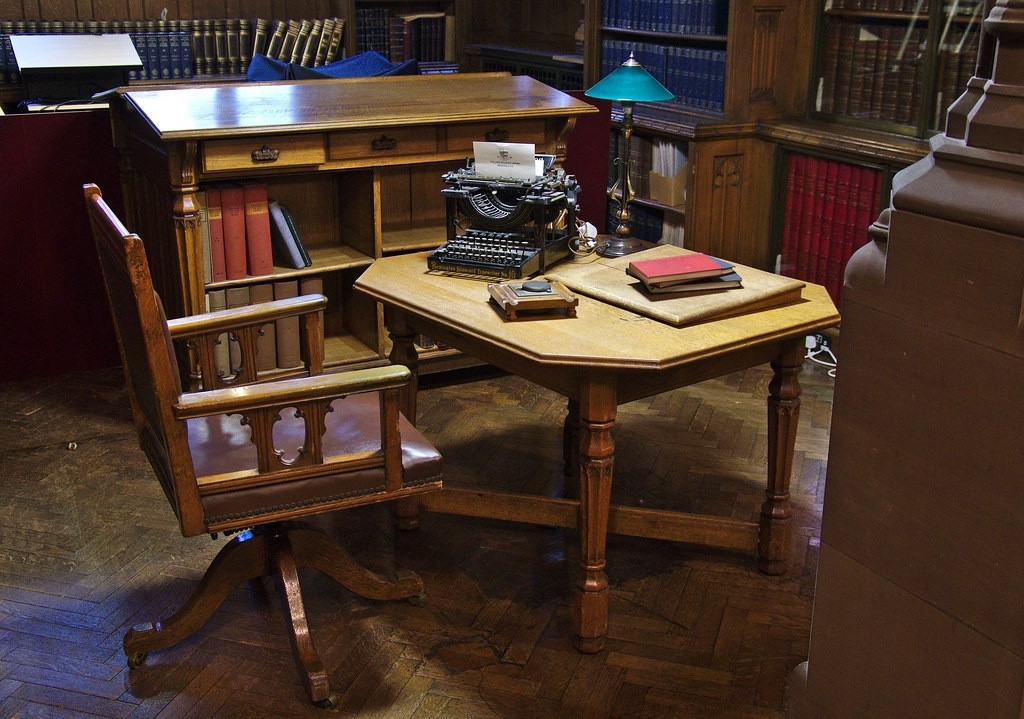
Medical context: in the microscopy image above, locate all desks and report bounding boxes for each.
[348,234,846,658]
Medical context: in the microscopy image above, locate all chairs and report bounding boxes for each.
[79,177,454,707]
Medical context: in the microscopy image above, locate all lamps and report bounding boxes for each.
[592,54,677,261]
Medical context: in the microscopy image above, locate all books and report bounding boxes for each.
[194,182,324,382]
[626,252,742,294]
[357,9,464,75]
[461,44,465,44]
[0,19,347,84]
[599,0,728,249]
[780,0,996,317]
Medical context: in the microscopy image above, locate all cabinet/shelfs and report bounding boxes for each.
[111,68,601,396]
[756,0,997,357]
[585,0,817,258]
